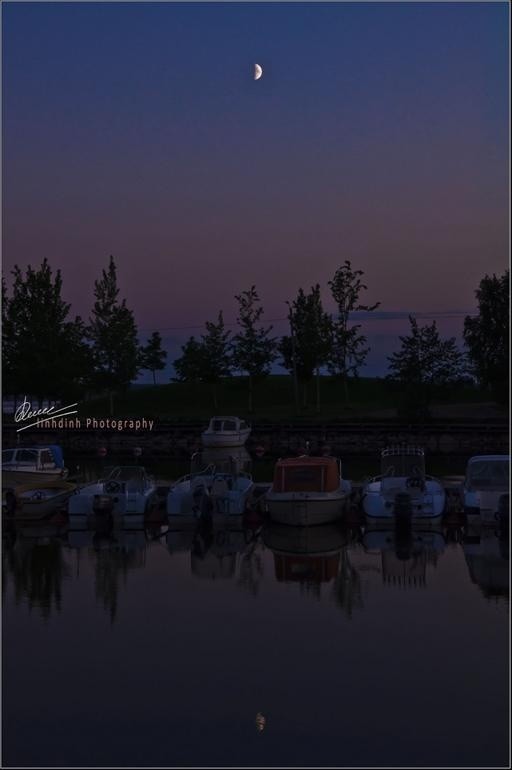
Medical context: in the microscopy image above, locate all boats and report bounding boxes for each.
[1,443,510,534]
[200,415,252,448]
[1,526,508,632]
